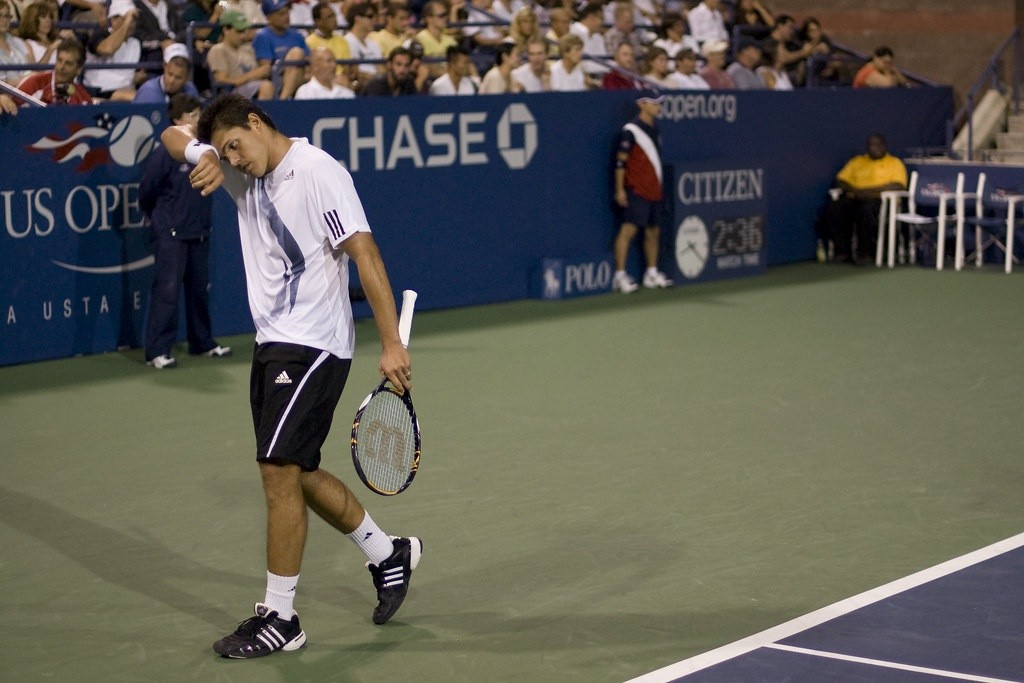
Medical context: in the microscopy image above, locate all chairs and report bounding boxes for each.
[828,169,1024,276]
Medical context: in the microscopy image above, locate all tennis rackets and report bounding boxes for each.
[351,291,420,497]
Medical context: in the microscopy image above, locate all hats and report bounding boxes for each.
[220,10,251,30]
[702,39,728,54]
[107,0,136,19]
[403,40,423,57]
[263,0,288,15]
[164,43,189,64]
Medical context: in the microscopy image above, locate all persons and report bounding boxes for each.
[834,134,908,265]
[610,87,674,293]
[0,0,909,116]
[136,94,232,371]
[161,93,422,659]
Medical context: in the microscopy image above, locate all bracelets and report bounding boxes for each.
[184,139,221,164]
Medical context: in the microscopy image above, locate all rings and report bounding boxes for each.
[405,371,410,376]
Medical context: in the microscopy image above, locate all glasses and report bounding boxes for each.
[362,13,376,20]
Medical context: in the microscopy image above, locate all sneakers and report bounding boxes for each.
[612,274,639,295]
[145,353,177,370]
[644,271,673,287]
[202,346,232,358]
[213,601,308,658]
[364,534,422,623]
